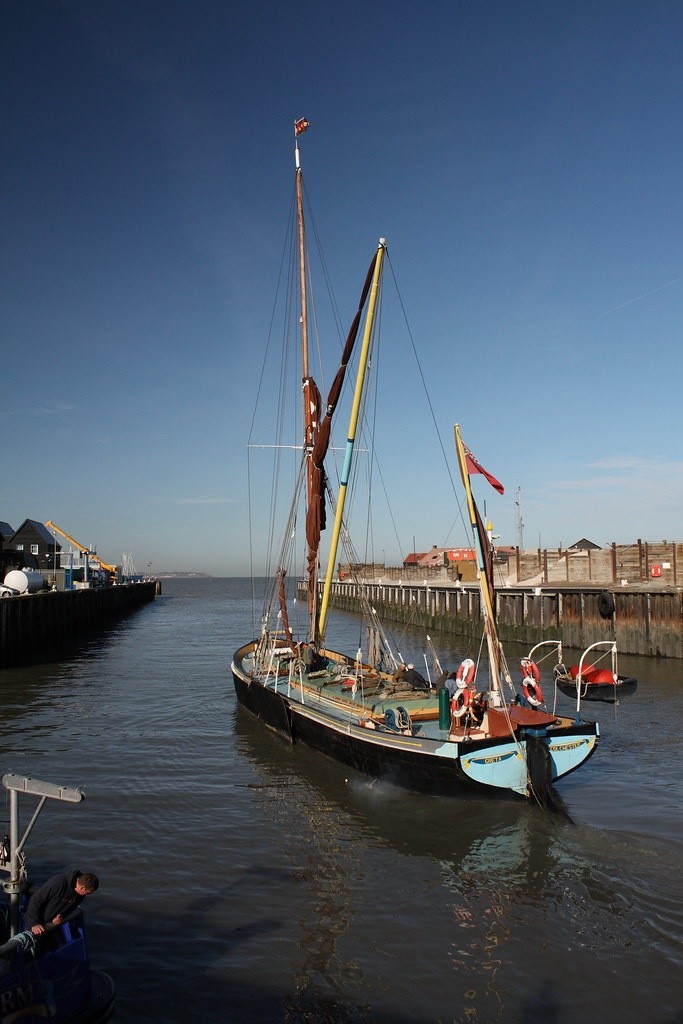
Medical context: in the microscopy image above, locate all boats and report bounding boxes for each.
[0,515,162,666]
[553,664,638,704]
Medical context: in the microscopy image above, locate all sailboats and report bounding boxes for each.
[227,115,602,805]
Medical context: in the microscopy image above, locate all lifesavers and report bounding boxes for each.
[520,656,540,685]
[522,677,543,707]
[455,659,475,687]
[450,689,470,717]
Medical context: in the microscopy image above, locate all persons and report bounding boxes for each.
[436,670,449,695]
[404,663,428,688]
[392,663,407,683]
[444,672,459,698]
[25,869,99,962]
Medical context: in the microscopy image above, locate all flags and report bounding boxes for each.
[461,440,505,495]
[294,117,307,137]
